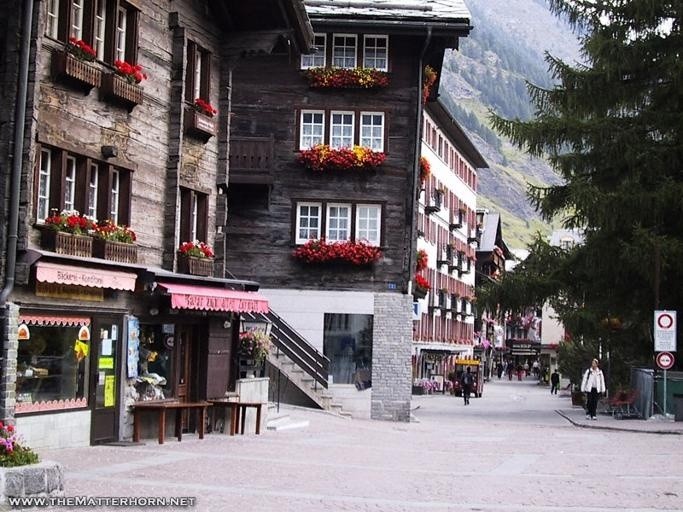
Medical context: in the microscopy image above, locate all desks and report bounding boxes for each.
[127,399,264,444]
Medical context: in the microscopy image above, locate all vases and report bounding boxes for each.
[412,284,428,298]
[185,113,215,144]
[179,255,213,277]
[40,228,137,266]
[105,74,142,106]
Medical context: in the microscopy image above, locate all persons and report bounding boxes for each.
[459,365,475,405]
[549,368,559,394]
[580,357,606,420]
[495,362,503,380]
[517,366,523,381]
[523,359,530,376]
[508,363,513,381]
[532,359,538,376]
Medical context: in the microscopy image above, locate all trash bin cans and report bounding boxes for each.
[629,367,683,422]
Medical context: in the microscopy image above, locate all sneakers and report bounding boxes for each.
[467,399,469,405]
[584,415,597,420]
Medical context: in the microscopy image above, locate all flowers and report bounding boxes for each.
[238,326,273,362]
[35,205,136,245]
[187,97,218,118]
[60,37,143,88]
[417,156,431,187]
[307,66,391,90]
[413,249,431,289]
[289,239,385,267]
[178,240,215,258]
[297,140,385,174]
[421,64,436,104]
